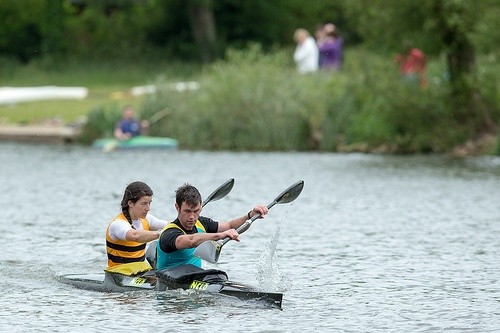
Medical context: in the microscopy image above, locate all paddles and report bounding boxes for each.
[103,106,173,152]
[194,179,304,263]
[144,179,236,258]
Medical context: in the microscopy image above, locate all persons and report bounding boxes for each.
[394,48,428,88]
[105,181,173,280]
[292,23,343,76]
[113,105,149,141]
[155,183,270,277]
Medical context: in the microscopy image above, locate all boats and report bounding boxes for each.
[54,268,284,310]
[92,136,179,155]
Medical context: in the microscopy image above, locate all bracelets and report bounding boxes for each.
[248,210,252,219]
